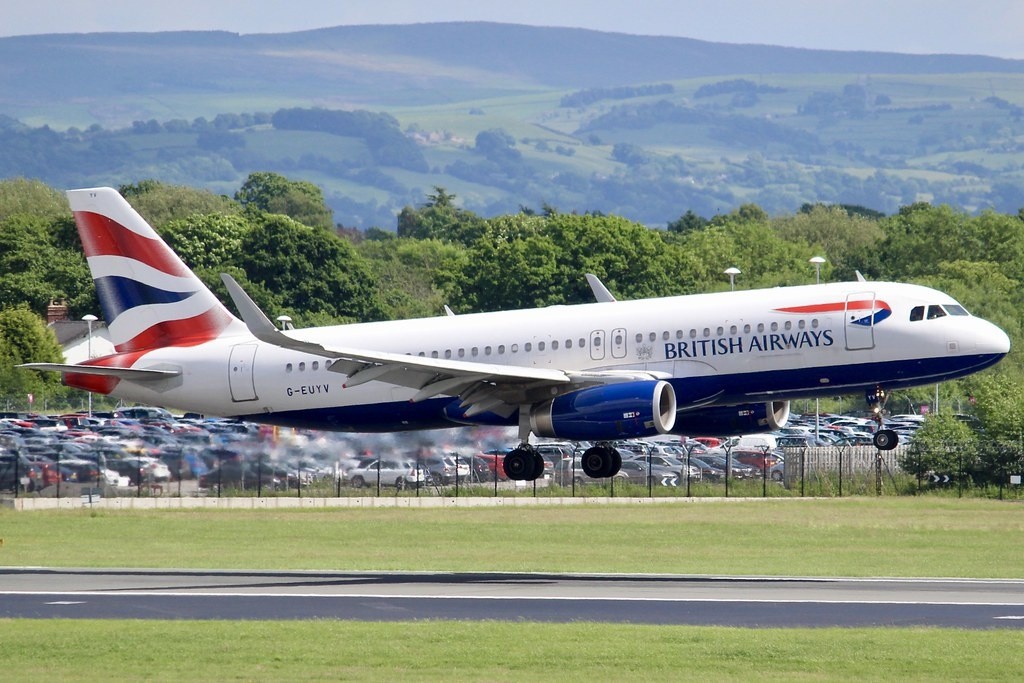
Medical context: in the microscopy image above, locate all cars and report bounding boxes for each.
[0,406,989,490]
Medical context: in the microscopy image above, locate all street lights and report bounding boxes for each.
[809,257,827,284]
[724,268,741,292]
[81,314,98,417]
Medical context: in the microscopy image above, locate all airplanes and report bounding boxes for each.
[15,186,1010,481]
